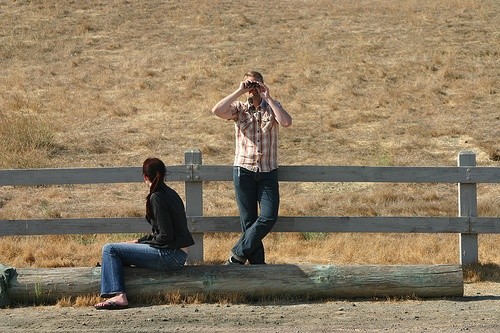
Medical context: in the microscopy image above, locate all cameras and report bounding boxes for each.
[248,82,260,88]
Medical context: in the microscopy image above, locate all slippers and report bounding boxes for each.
[94,300,129,310]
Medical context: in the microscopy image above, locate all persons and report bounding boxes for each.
[93,157,196,309]
[212,70,293,266]
